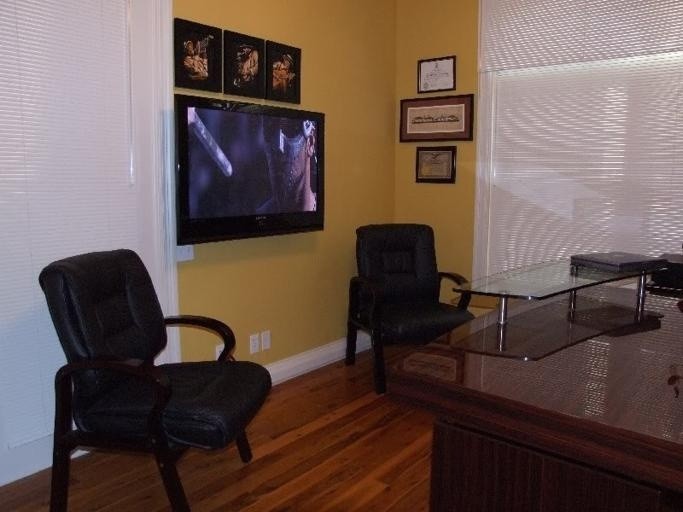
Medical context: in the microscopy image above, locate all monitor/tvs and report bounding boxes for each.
[174,94,324,247]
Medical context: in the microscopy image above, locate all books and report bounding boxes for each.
[570,248,667,272]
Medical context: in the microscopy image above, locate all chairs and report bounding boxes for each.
[345,223,472,394]
[38,246,274,512]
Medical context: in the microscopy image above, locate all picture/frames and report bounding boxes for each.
[174,18,223,92]
[397,94,474,142]
[263,38,301,104]
[417,54,457,93]
[222,28,264,100]
[415,145,456,183]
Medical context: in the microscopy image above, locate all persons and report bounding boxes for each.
[232,42,259,88]
[182,40,209,81]
[256,116,317,214]
[271,53,296,96]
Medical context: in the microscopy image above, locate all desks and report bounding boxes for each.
[398,271,683,510]
